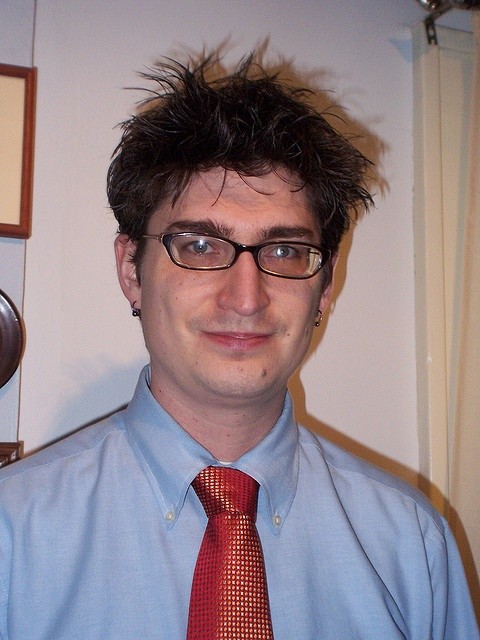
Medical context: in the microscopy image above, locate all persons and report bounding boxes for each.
[0,55,477,639]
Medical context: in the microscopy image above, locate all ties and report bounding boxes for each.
[186,465,274,640]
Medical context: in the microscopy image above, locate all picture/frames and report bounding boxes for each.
[0,61,38,239]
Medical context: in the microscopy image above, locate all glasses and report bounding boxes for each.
[139,232,331,279]
